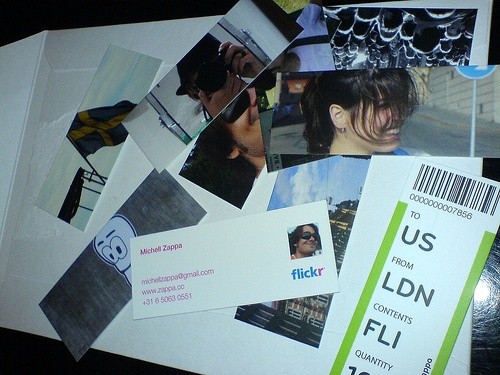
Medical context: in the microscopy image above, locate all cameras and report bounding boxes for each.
[187,46,244,98]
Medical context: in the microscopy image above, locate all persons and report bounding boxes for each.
[287,222,322,260]
[300,67,413,155]
[176,33,266,137]
[198,81,267,176]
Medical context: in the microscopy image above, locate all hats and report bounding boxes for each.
[176,33,223,96]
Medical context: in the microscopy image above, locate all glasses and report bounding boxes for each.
[301,232,319,241]
[220,69,268,122]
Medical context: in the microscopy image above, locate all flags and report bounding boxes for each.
[66,100,137,160]
